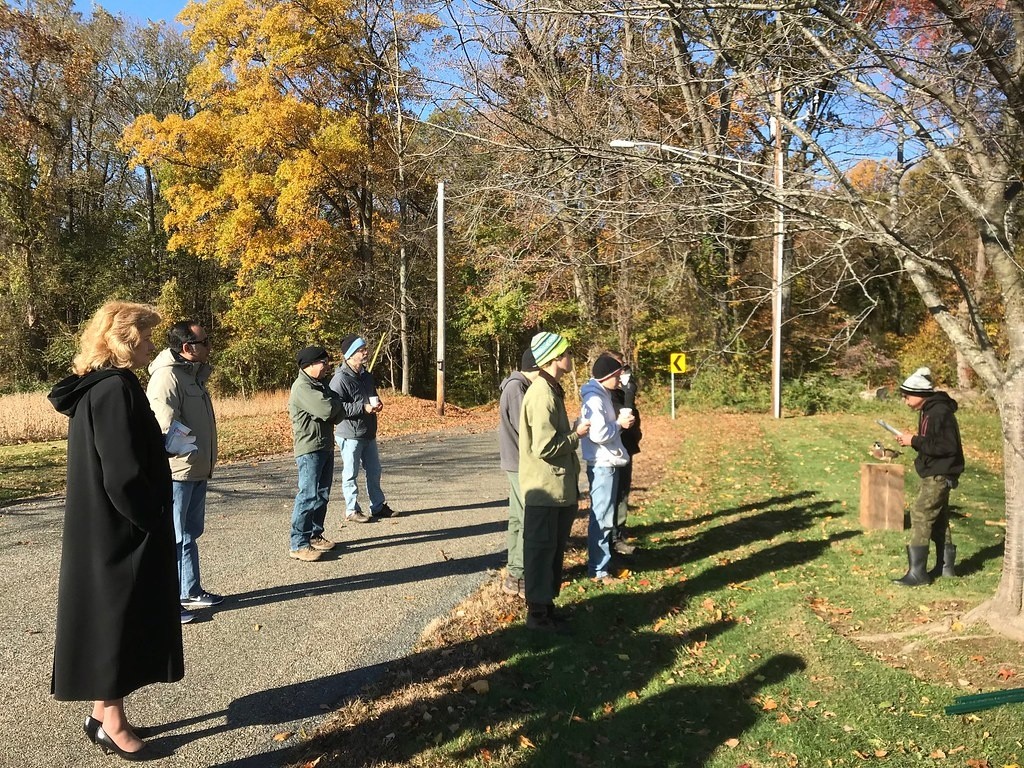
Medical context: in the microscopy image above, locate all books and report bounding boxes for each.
[875,419,903,437]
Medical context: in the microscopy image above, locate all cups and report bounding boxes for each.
[165,419,192,450]
[619,407,632,416]
[369,396,378,406]
[619,374,631,386]
[577,417,590,428]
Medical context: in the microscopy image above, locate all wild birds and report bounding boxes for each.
[873,441,904,464]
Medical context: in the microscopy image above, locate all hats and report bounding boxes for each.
[899,366,935,397]
[341,333,366,360]
[521,347,540,371]
[531,332,569,368]
[592,356,622,381]
[297,347,328,369]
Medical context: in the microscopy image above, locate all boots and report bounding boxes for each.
[891,544,933,587]
[927,544,960,580]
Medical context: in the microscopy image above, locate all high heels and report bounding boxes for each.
[83,715,103,745]
[95,724,162,762]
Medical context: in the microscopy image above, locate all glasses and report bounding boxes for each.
[186,337,210,347]
[316,360,328,365]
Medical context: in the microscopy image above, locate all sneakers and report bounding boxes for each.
[180,589,224,606]
[180,603,197,624]
[347,510,371,523]
[309,534,336,550]
[289,545,323,562]
[372,502,399,517]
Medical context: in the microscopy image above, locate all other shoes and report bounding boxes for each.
[611,538,638,556]
[590,574,624,587]
[518,578,525,599]
[524,611,566,633]
[550,603,570,621]
[501,570,519,595]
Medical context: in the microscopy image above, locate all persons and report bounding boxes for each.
[612,359,642,544]
[581,355,637,578]
[146,321,225,623]
[289,348,346,562]
[48,301,185,760]
[520,332,592,628]
[329,334,400,523]
[498,348,540,600]
[893,367,965,586]
